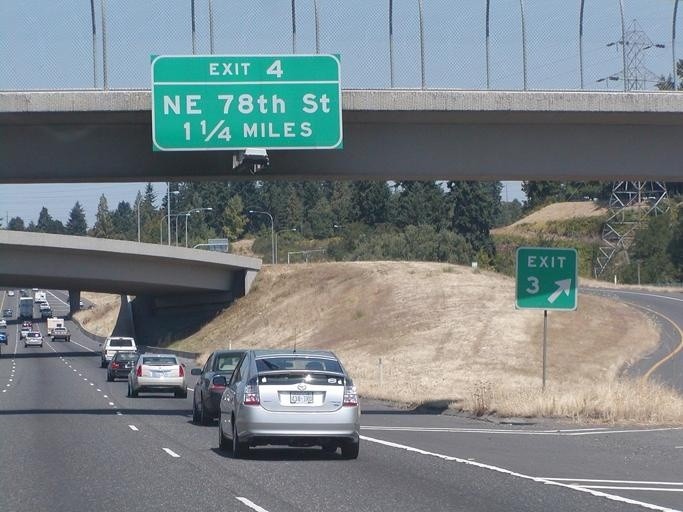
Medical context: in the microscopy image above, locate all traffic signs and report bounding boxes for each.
[151,56,342,153]
[515,246,576,310]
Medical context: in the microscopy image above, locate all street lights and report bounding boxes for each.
[334,224,345,229]
[135,189,309,266]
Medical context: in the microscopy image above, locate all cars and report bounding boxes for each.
[217,348,360,461]
[191,347,243,424]
[0,286,187,398]
